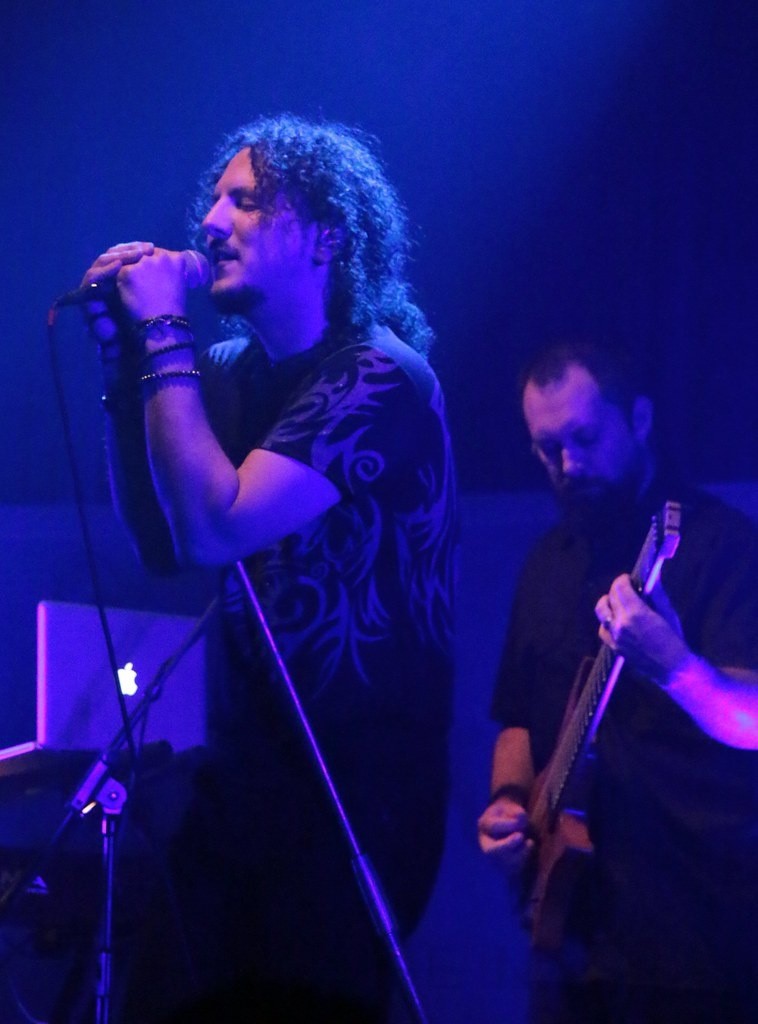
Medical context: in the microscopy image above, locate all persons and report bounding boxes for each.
[477,327,758,1023]
[76,113,458,1024]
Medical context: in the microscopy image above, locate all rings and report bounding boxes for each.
[603,617,612,630]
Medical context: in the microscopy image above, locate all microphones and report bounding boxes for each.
[56,250,210,306]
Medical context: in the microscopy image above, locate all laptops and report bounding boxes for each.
[0,601,204,776]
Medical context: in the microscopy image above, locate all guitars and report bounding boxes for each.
[512,496,683,955]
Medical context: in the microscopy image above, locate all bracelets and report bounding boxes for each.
[138,340,192,362]
[140,367,202,380]
[135,313,192,331]
[489,783,530,809]
[96,337,139,413]
